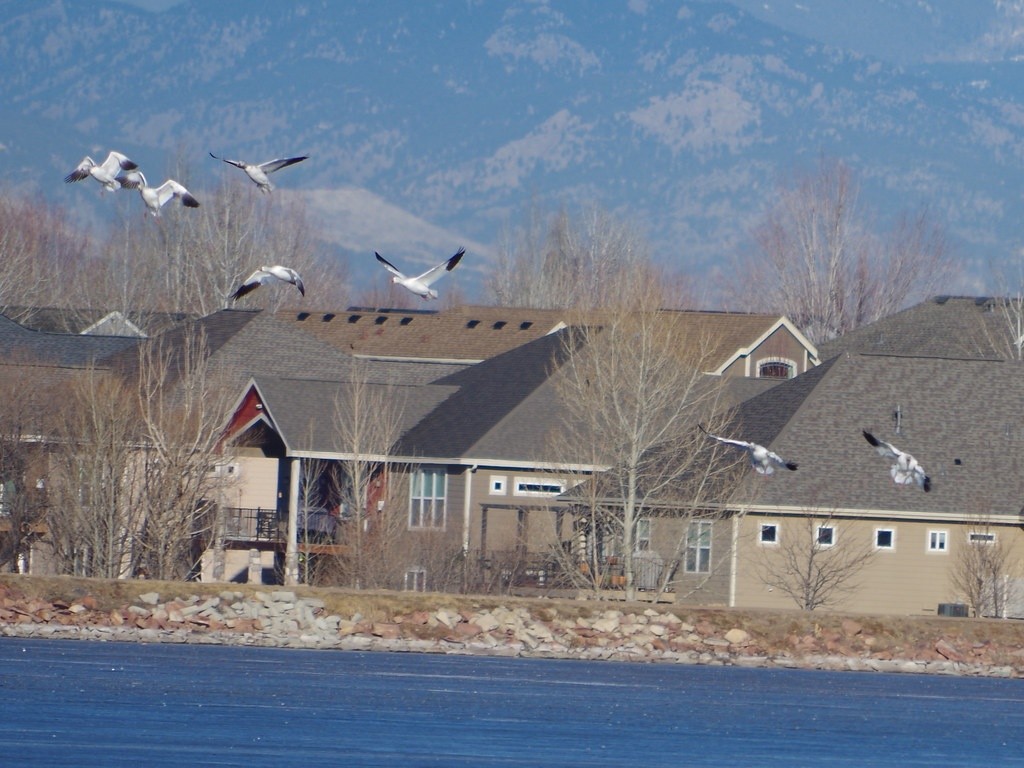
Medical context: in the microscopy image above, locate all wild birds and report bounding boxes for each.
[230,265,304,301]
[207,150,310,195]
[63,151,138,191]
[115,172,200,218]
[695,422,800,476]
[861,430,931,494]
[373,246,467,301]
